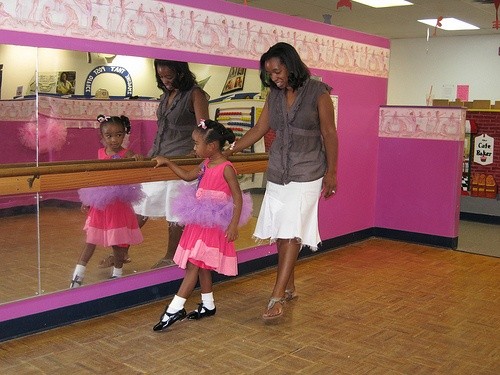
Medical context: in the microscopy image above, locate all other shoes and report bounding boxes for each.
[153,306,187,332]
[187,303,216,320]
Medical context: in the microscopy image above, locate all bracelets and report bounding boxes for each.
[228,140,237,157]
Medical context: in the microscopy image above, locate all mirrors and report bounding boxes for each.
[0,44,322,306]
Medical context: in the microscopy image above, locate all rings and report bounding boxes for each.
[331,189,336,194]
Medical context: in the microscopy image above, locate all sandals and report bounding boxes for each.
[98,254,131,268]
[285,286,296,300]
[149,259,174,269]
[263,297,285,321]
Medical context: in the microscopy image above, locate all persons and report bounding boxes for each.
[97,59,210,282]
[224,68,244,90]
[151,118,255,332]
[57,72,72,95]
[70,113,145,291]
[219,42,339,321]
